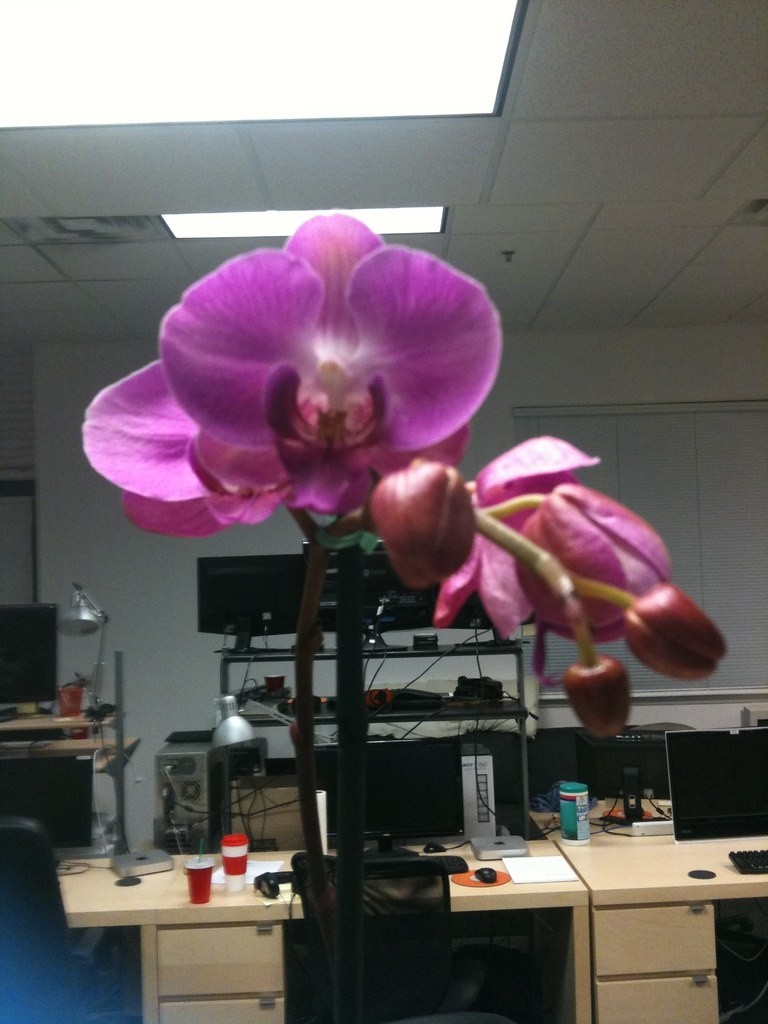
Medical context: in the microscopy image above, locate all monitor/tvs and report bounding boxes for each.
[1,756,93,846]
[196,541,536,652]
[0,603,59,704]
[312,737,465,860]
[664,726,768,844]
[576,729,671,826]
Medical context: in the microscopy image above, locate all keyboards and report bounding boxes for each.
[729,849,768,875]
[364,856,468,880]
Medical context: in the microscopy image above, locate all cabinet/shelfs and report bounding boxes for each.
[212,640,533,840]
[1,710,141,857]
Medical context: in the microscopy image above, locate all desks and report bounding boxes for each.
[529,795,768,1024]
[52,839,594,1024]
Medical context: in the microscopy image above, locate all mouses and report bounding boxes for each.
[475,867,497,883]
[423,841,446,853]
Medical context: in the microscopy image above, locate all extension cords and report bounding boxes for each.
[632,821,674,835]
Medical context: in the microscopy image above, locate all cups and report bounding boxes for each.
[264,676,285,690]
[221,834,249,891]
[185,857,215,904]
[60,689,82,716]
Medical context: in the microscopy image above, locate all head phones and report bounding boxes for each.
[255,871,301,898]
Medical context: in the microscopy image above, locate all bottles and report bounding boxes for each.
[560,783,591,846]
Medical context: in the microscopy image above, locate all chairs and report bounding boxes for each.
[0,820,107,1024]
[522,727,625,814]
[288,850,549,1024]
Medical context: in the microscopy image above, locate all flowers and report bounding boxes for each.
[85,208,732,740]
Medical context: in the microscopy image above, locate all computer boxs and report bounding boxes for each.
[154,740,223,855]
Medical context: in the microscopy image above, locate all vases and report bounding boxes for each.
[56,687,83,718]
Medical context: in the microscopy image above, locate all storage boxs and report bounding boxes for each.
[228,773,309,852]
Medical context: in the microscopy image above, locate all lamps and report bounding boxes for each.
[57,580,109,703]
